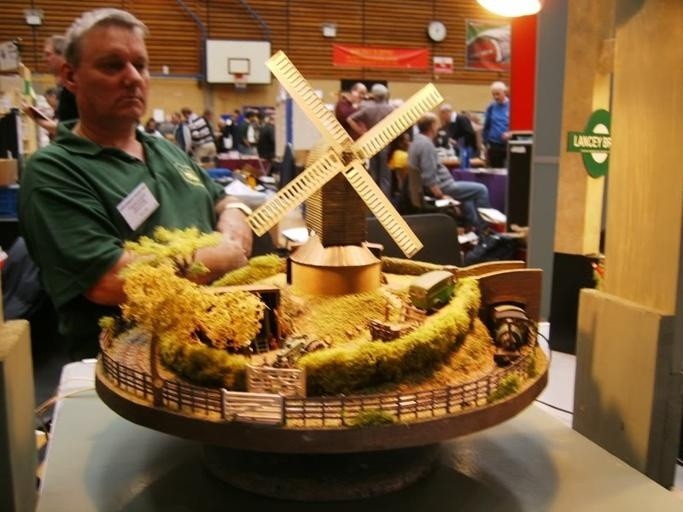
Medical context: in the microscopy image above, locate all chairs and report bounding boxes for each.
[403,165,462,222]
[353,214,461,265]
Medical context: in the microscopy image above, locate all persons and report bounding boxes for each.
[21,34,78,146]
[18,8,253,362]
[336,78,512,239]
[143,101,276,178]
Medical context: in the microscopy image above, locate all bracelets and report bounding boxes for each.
[224,202,253,217]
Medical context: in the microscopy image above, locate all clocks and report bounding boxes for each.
[427,21,447,44]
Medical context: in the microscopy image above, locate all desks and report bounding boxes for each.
[452,167,507,208]
[36,357,681,510]
[212,152,257,170]
